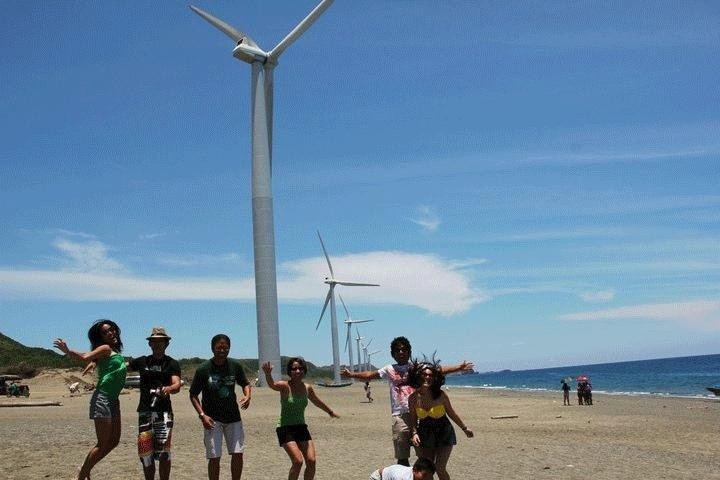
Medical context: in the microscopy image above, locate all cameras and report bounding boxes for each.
[149,386,164,408]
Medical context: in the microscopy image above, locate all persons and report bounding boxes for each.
[123,327,182,480]
[369,457,436,480]
[407,349,474,480]
[363,382,373,403]
[561,379,592,406]
[339,336,476,468]
[189,334,252,480]
[51,318,127,480]
[261,357,340,480]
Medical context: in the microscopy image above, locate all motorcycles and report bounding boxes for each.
[6,388,20,398]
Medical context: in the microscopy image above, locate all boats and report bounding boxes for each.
[315,382,354,388]
[707,385,720,397]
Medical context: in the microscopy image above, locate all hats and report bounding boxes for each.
[145,327,172,341]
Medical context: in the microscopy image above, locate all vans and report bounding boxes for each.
[125,375,141,389]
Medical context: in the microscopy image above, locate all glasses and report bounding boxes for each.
[290,366,304,371]
[394,346,409,353]
[100,325,116,336]
[419,371,434,378]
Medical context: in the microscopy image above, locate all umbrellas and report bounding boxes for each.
[576,375,591,382]
[562,376,573,382]
